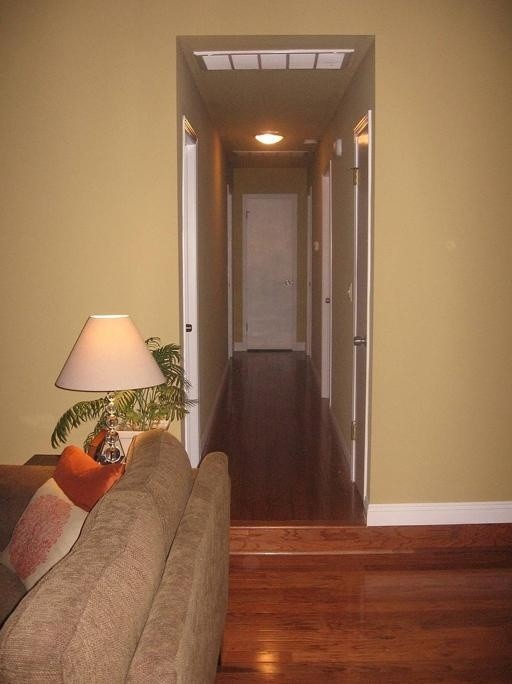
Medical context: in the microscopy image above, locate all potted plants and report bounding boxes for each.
[51,336,200,458]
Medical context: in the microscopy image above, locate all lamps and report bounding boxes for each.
[55,314,167,462]
[255,133,284,144]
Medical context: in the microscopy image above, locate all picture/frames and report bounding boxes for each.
[88,430,107,460]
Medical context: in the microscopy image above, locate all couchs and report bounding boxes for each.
[0,428,231,683]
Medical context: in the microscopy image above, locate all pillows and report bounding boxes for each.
[0,444,125,593]
